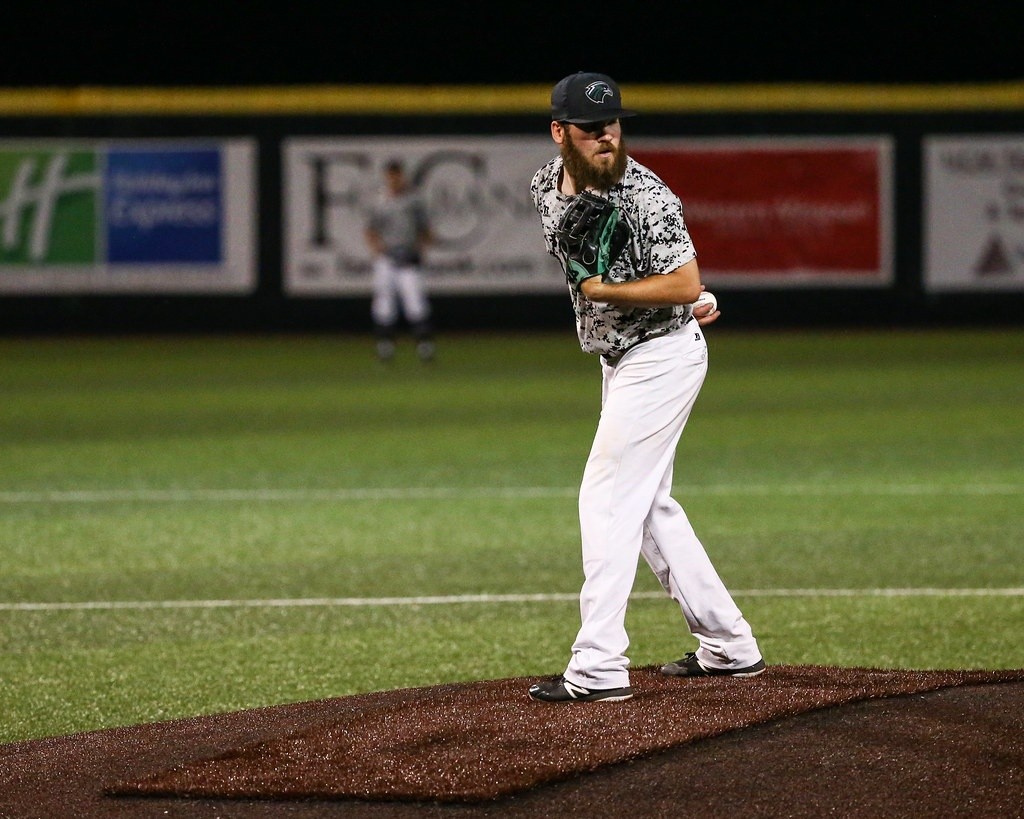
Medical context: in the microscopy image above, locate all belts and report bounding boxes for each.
[601,332,663,359]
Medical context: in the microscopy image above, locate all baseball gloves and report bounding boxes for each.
[556,191,631,290]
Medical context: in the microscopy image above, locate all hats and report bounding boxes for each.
[551,71,637,124]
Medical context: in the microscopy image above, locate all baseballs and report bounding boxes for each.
[691,291,718,317]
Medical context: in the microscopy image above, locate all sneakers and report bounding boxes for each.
[528,673,634,702]
[660,651,766,678]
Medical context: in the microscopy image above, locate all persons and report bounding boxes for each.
[361,160,444,356]
[528,73,764,704]
